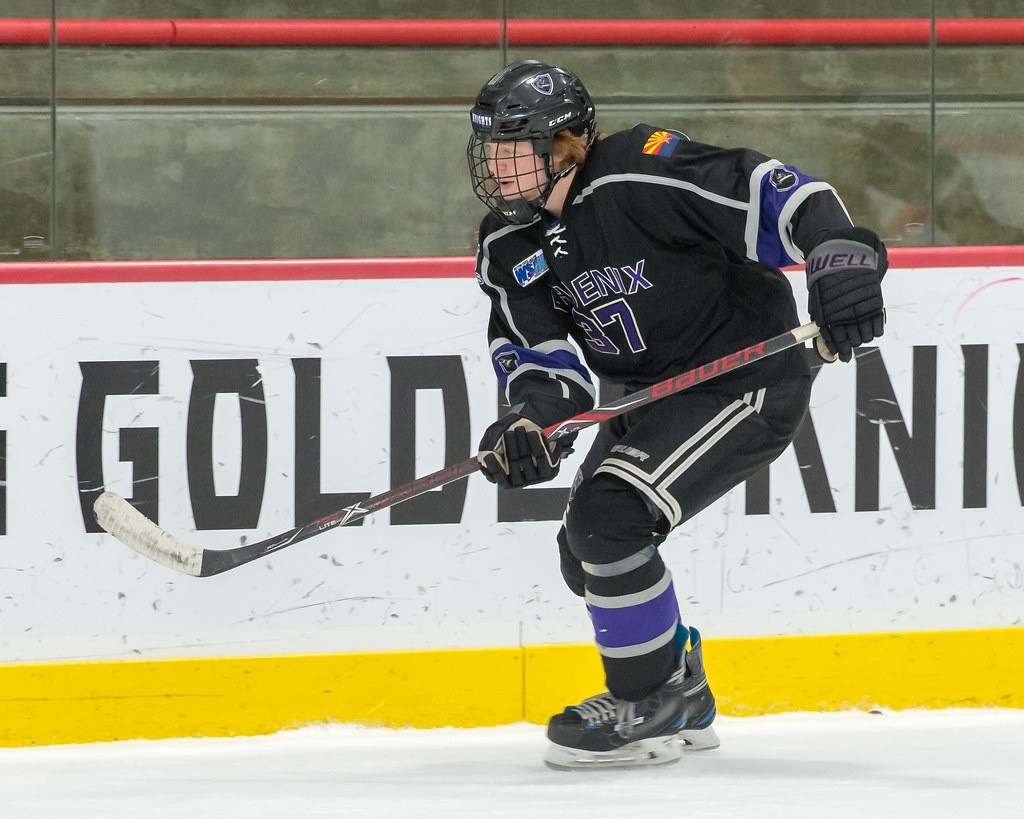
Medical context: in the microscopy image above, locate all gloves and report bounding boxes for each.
[477,390,580,491]
[804,226,890,364]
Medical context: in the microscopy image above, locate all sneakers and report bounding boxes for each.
[542,625,720,770]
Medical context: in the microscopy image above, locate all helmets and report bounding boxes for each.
[466,59,597,226]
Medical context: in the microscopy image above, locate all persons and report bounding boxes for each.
[465,59,889,771]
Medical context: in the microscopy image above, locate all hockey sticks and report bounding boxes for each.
[94,320,821,579]
